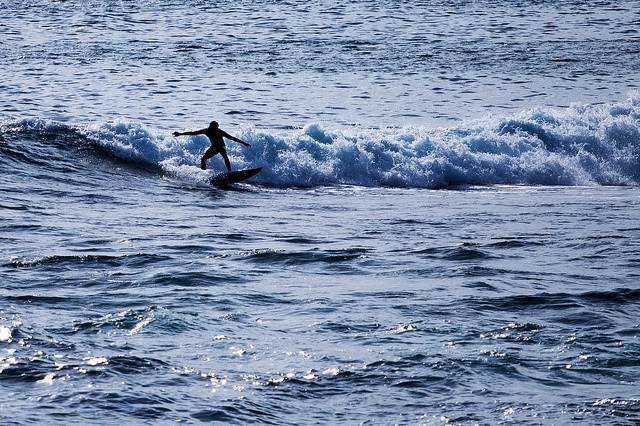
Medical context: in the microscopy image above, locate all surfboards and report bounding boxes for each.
[210,167,263,186]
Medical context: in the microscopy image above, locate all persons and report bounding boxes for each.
[172,121,251,172]
